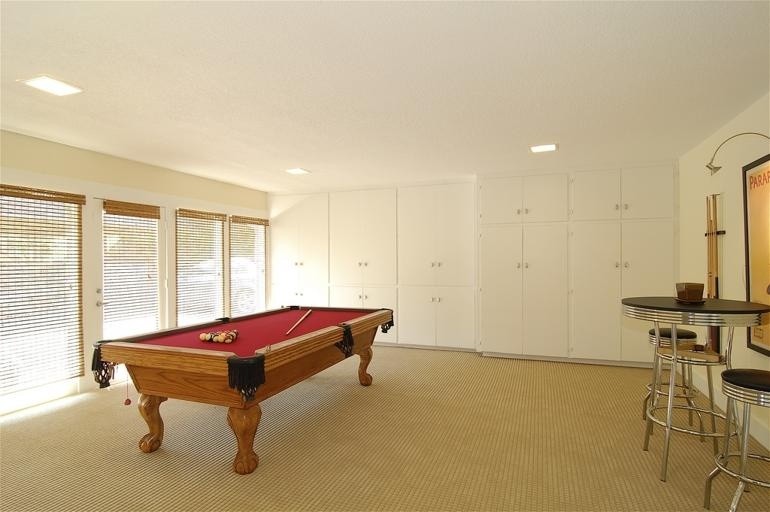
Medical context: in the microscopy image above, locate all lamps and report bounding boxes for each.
[704,132,770,176]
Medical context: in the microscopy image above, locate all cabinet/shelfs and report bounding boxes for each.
[396,179,479,287]
[567,217,682,368]
[264,191,328,284]
[328,283,396,349]
[477,169,570,226]
[566,159,681,220]
[475,220,569,365]
[327,187,398,287]
[267,283,331,311]
[396,283,480,354]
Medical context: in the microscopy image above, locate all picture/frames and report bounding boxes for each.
[739,153,769,358]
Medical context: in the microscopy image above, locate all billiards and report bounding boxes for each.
[198,330,239,344]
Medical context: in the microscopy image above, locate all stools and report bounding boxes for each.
[639,326,708,447]
[700,362,769,512]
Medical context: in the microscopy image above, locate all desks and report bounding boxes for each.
[619,292,769,495]
[87,304,399,477]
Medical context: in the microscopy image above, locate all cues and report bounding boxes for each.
[285,309,312,333]
[702,193,717,357]
